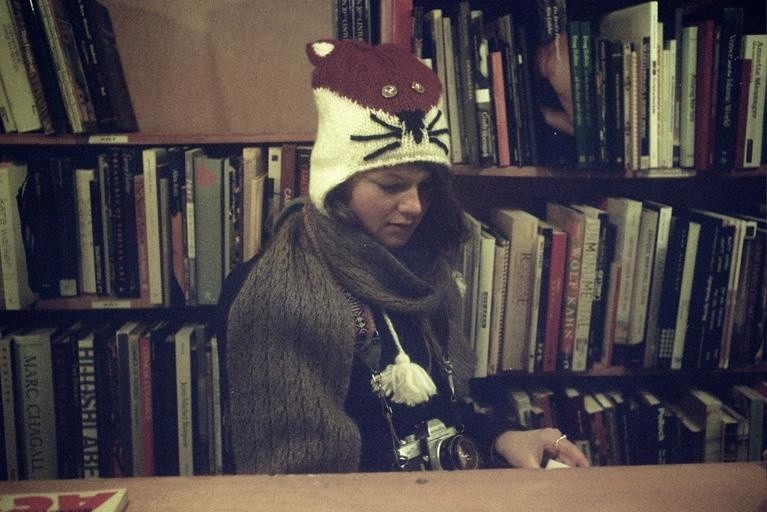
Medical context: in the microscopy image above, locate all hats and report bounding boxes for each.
[306,39,467,407]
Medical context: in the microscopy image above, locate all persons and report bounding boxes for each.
[216,39,595,475]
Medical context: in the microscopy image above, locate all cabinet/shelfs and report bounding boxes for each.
[3,4,765,382]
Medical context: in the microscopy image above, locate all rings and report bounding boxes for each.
[556,434,567,448]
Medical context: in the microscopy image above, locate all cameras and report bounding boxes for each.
[393,417,480,470]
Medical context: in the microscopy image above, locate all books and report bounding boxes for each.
[332,2,765,170]
[506,385,766,467]
[2,1,140,135]
[1,314,237,482]
[457,197,766,379]
[0,489,129,512]
[0,146,309,310]
